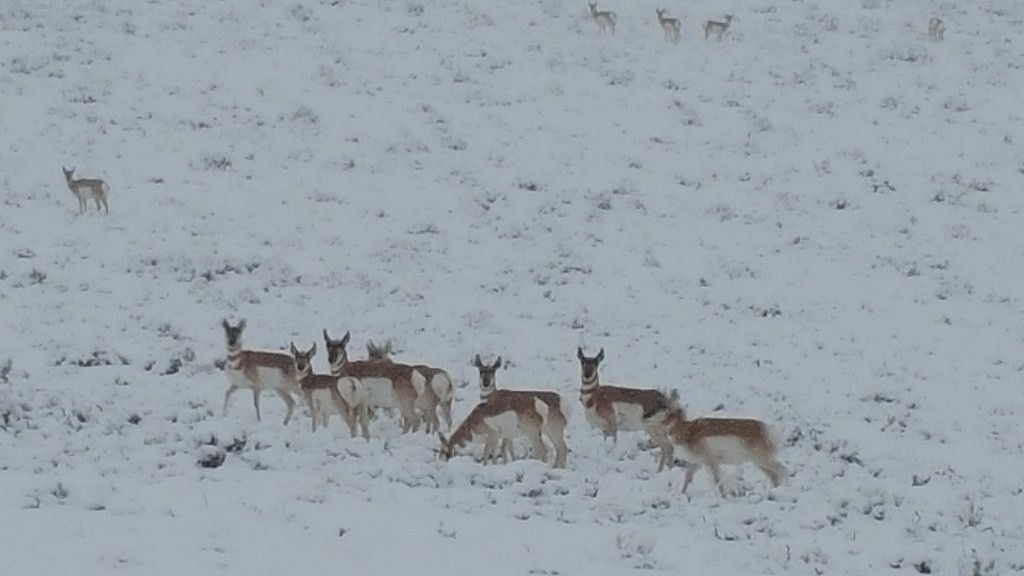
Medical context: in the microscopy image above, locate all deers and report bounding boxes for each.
[62,166,110,214]
[588,1,617,34]
[704,14,736,41]
[437,354,569,469]
[655,8,683,46]
[641,388,783,500]
[577,347,683,476]
[221,320,452,443]
